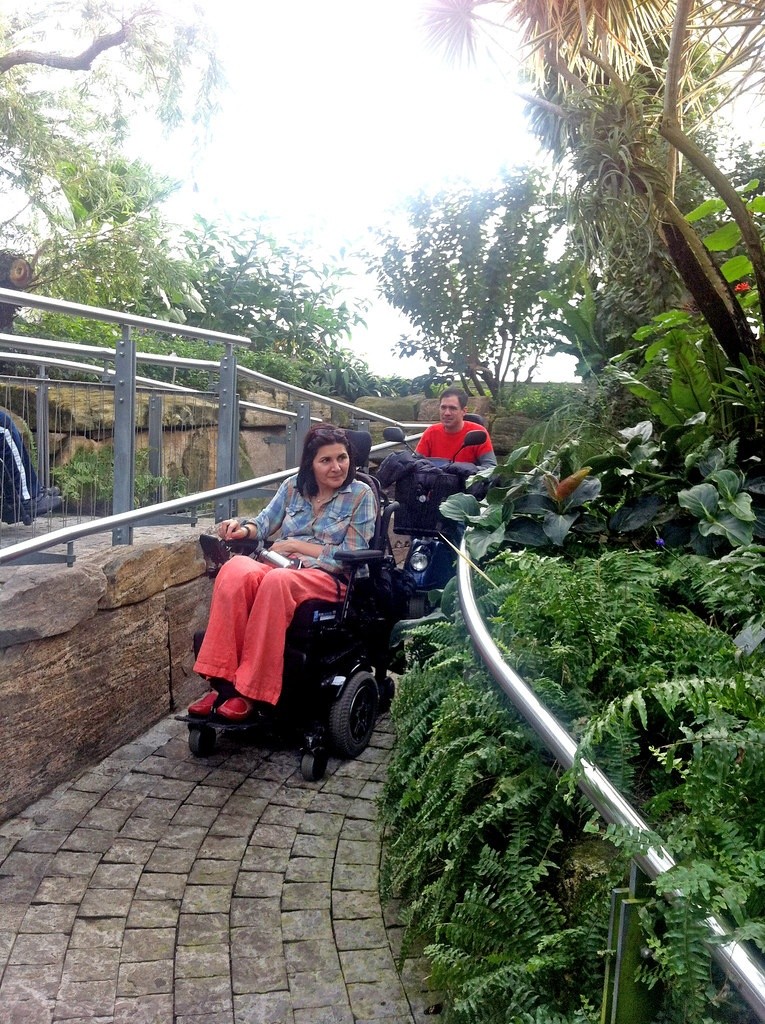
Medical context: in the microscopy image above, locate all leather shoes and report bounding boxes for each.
[216,697,252,719]
[187,692,217,714]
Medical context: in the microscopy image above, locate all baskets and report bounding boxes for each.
[392,473,460,540]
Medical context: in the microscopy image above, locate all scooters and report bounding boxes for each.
[382,414,487,618]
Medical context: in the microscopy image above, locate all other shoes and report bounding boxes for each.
[22,494,63,516]
[40,486,59,496]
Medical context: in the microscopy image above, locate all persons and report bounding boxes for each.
[411,387,497,592]
[187,422,379,719]
[0,411,64,523]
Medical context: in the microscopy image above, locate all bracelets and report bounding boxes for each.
[235,524,252,540]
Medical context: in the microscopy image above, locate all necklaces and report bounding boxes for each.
[314,493,331,504]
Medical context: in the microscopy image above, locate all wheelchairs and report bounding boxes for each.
[174,431,430,783]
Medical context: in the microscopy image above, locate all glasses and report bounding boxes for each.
[306,428,346,446]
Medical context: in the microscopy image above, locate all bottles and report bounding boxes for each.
[255,547,294,568]
